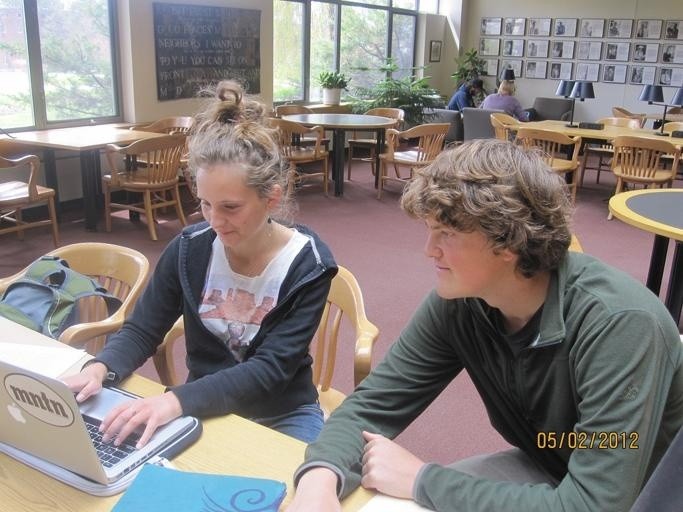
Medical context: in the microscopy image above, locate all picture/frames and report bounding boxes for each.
[477,17,683,88]
[430,40,442,62]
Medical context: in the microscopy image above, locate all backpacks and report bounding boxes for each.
[0,255,123,341]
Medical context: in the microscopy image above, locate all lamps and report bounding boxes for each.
[638,84,683,136]
[555,80,595,128]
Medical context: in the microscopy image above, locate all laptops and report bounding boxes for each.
[0,361,195,484]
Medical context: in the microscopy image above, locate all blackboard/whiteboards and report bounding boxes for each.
[153,4,261,101]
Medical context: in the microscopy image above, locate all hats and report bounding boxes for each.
[470,79,487,91]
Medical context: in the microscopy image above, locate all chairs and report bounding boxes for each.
[277,106,330,180]
[376,122,451,200]
[422,97,683,221]
[0,155,61,249]
[347,108,405,180]
[130,117,198,214]
[153,264,380,423]
[102,134,188,241]
[263,118,329,204]
[0,242,151,357]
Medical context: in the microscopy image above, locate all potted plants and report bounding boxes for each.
[320,72,352,105]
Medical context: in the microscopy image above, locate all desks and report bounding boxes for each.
[609,188,683,334]
[0,373,379,512]
[280,114,398,197]
[0,123,170,232]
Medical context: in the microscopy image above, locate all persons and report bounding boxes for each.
[447,78,483,116]
[283,136,682,511]
[478,78,529,122]
[64,78,339,450]
